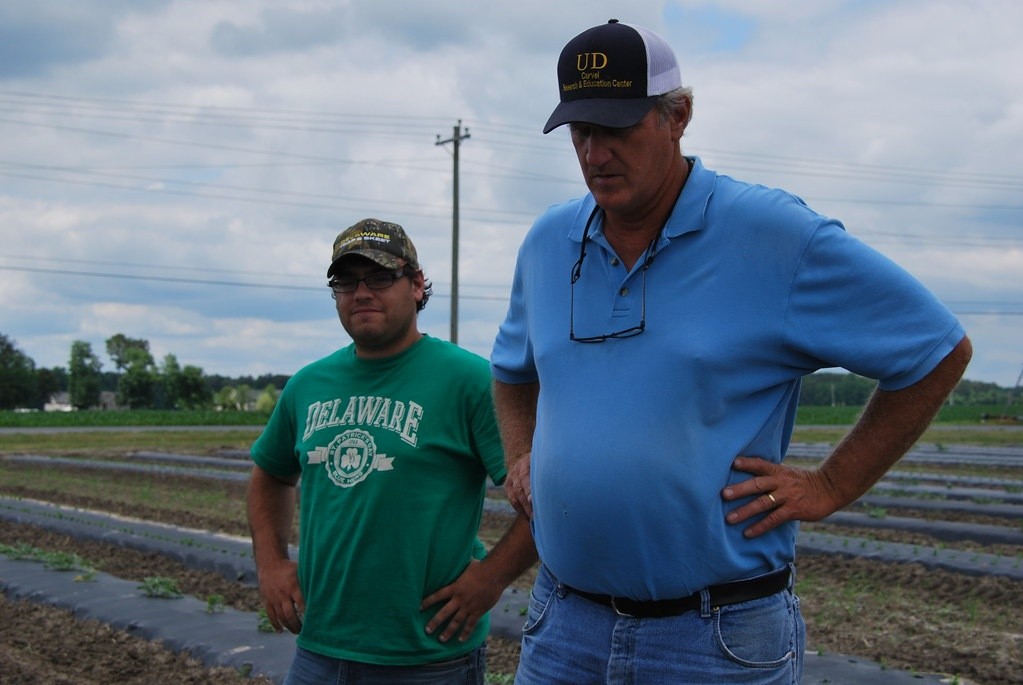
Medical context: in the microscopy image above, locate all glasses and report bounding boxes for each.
[329,270,406,294]
[569,237,657,344]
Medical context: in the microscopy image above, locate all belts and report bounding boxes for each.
[563,563,792,620]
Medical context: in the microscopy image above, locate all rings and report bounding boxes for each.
[768,493,776,508]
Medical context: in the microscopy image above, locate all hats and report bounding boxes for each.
[326,218,420,279]
[542,18,682,135]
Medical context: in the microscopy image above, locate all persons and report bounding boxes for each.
[490,19,972,685]
[247,219,539,685]
